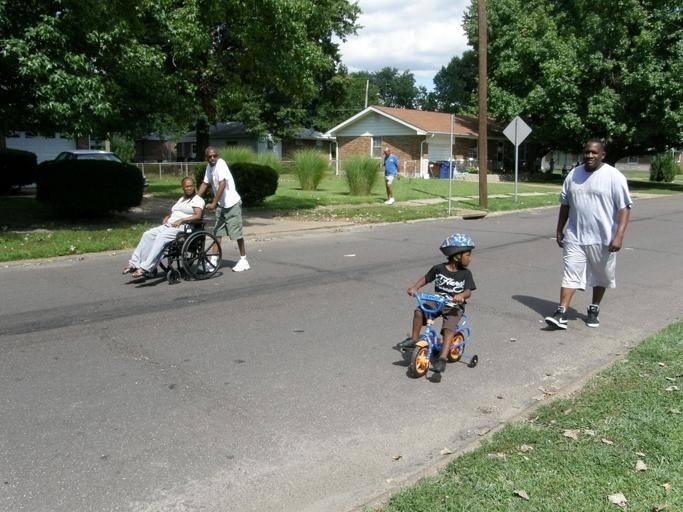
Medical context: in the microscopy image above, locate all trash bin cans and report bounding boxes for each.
[428,160,455,179]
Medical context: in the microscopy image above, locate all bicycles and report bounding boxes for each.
[400,290,478,378]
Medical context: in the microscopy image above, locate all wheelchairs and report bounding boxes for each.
[130,205,223,284]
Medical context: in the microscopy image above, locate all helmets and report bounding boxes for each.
[439,232,475,257]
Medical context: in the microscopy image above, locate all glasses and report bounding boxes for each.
[208,153,218,158]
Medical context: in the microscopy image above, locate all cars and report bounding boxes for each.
[55,150,150,191]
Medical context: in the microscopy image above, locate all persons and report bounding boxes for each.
[120,177,206,280]
[401,233,475,374]
[196,146,252,273]
[380,148,401,205]
[544,140,632,331]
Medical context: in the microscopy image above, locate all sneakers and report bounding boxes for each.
[198,262,218,272]
[123,265,134,274]
[397,337,417,348]
[385,197,394,205]
[545,306,568,329]
[232,260,251,272]
[429,358,447,372]
[132,267,148,278]
[586,304,600,327]
